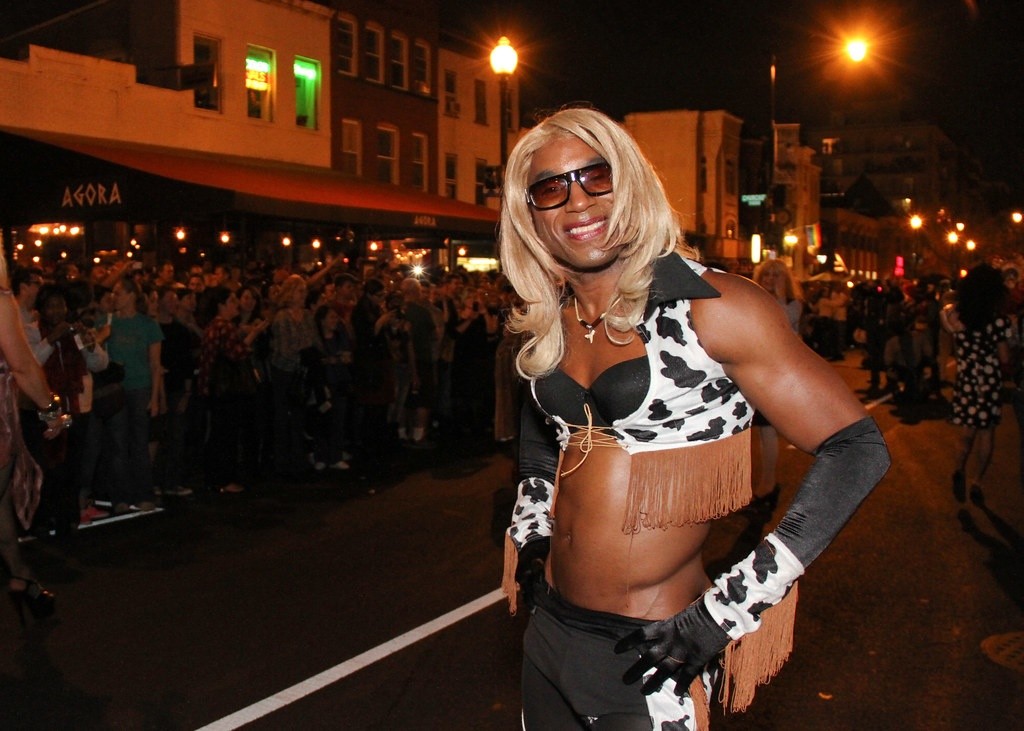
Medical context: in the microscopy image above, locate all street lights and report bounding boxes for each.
[486,34,521,177]
[767,31,867,265]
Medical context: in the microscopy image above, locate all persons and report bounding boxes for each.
[43,233,1024,635]
[0,286,56,629]
[499,107,892,731]
[11,268,43,306]
[24,282,110,526]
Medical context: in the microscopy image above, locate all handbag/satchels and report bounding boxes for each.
[77,374,93,413]
[81,332,109,372]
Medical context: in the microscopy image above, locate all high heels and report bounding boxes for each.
[8,576,57,628]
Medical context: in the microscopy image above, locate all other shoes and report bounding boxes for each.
[315,463,325,470]
[166,488,192,496]
[329,460,349,469]
[140,502,154,511]
[412,428,425,441]
[79,507,109,525]
[969,485,983,504]
[227,484,243,492]
[397,428,406,439]
[115,503,129,513]
[953,470,966,502]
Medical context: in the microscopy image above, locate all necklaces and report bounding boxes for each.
[574,296,621,344]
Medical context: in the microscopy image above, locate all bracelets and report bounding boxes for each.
[37,392,62,422]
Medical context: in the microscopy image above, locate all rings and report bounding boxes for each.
[47,427,56,432]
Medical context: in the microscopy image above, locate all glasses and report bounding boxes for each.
[375,293,385,298]
[524,162,613,211]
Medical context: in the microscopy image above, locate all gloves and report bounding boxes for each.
[515,536,550,610]
[613,587,732,696]
